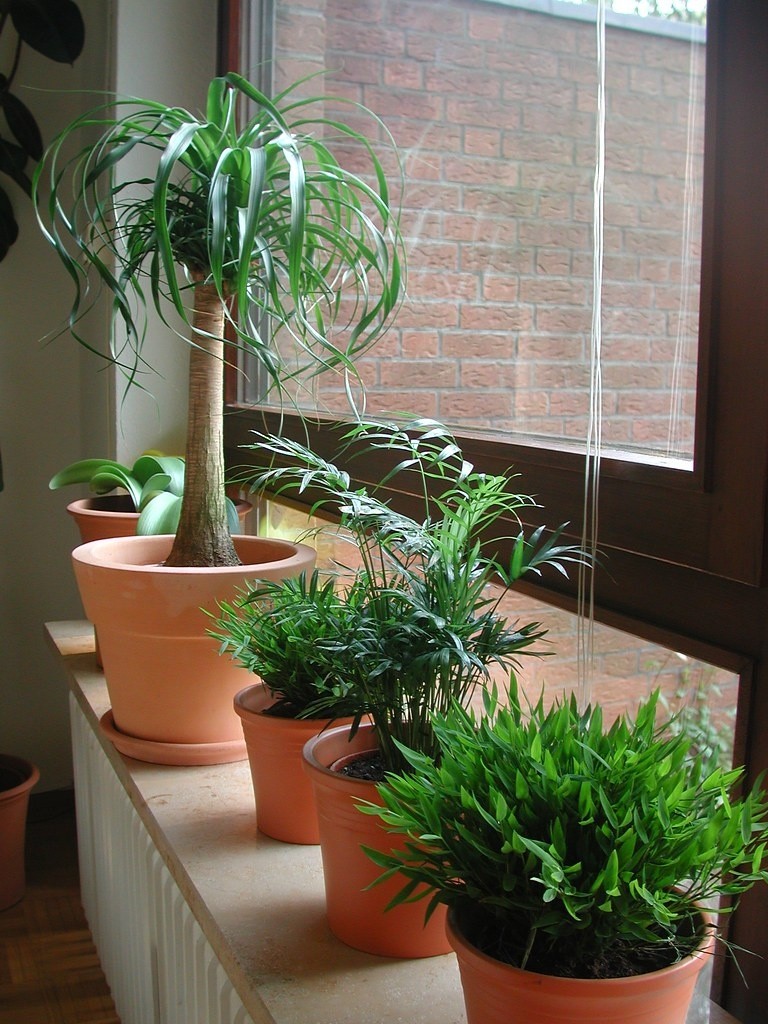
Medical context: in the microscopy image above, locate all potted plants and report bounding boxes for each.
[236,406,604,958]
[30,71,411,767]
[351,666,768,1024]
[49,456,240,536]
[201,567,375,842]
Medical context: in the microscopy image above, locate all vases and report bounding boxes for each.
[0,755,39,911]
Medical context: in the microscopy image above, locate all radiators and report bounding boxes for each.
[67,691,256,1024]
